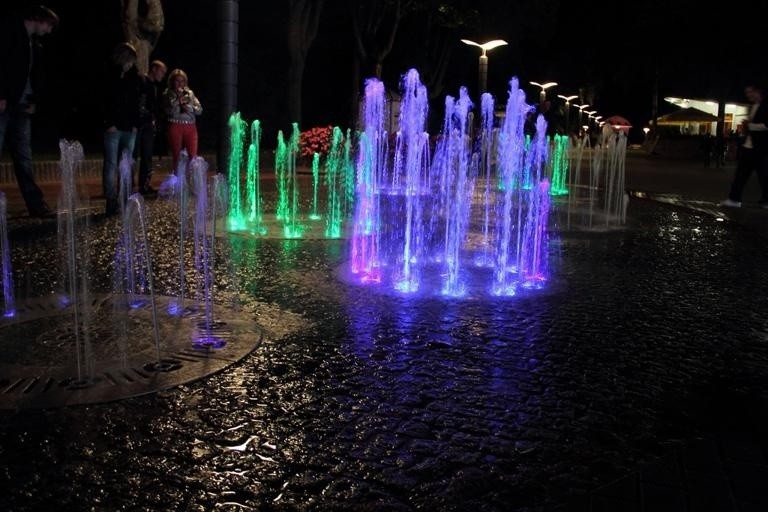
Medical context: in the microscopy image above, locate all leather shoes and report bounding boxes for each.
[28,207,57,218]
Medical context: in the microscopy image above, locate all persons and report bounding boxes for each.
[0,4,60,218]
[163,69,204,173]
[712,131,725,168]
[539,99,555,137]
[131,60,168,196]
[94,42,143,215]
[719,87,768,208]
[524,111,537,141]
[704,132,714,168]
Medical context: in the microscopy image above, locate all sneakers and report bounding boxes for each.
[138,186,159,197]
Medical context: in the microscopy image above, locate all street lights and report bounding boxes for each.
[643,128,650,139]
[461,39,507,92]
[574,104,605,136]
[531,81,557,113]
[559,95,577,135]
